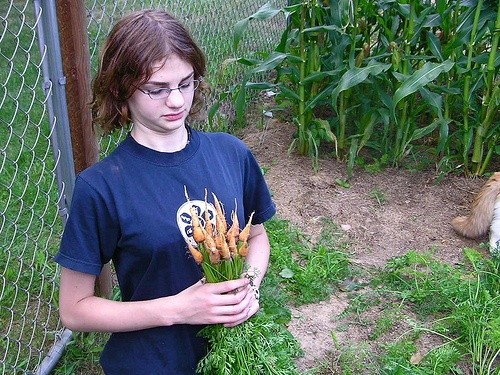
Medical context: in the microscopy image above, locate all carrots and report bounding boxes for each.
[183,186,255,266]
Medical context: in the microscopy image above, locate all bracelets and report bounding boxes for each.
[245,273,260,302]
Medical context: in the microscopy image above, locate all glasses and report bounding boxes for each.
[130,79,200,100]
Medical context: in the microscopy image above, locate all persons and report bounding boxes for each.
[55,10,277,372]
